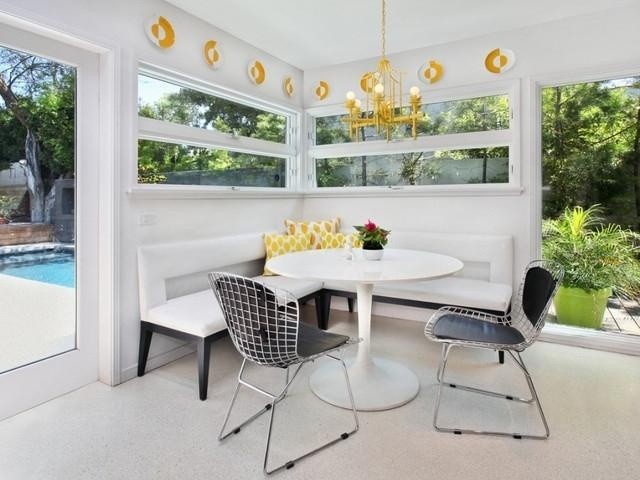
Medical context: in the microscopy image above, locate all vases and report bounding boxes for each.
[363,249,383,260]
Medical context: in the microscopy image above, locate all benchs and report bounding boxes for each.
[137,228,517,403]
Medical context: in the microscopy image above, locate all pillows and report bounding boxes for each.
[263,218,363,276]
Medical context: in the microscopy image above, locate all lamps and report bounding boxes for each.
[340,0,426,144]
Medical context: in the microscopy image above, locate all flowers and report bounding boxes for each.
[352,218,392,249]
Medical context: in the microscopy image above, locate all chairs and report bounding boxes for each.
[207,271,364,477]
[425,259,565,441]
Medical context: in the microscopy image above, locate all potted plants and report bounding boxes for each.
[543,203,639,329]
